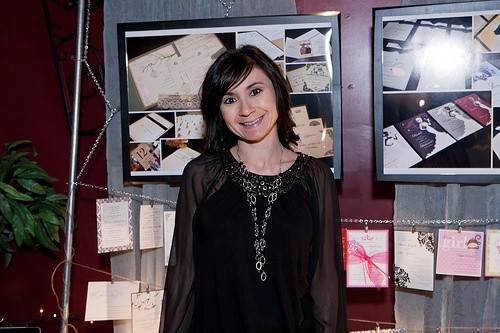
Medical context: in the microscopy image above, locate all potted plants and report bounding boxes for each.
[0,139,79,333]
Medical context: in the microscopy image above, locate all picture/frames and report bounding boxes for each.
[373,0,500,183]
[116,11,342,181]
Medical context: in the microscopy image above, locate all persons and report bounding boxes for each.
[157,43,348,333]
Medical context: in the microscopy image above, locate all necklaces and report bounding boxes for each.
[235,142,283,282]
